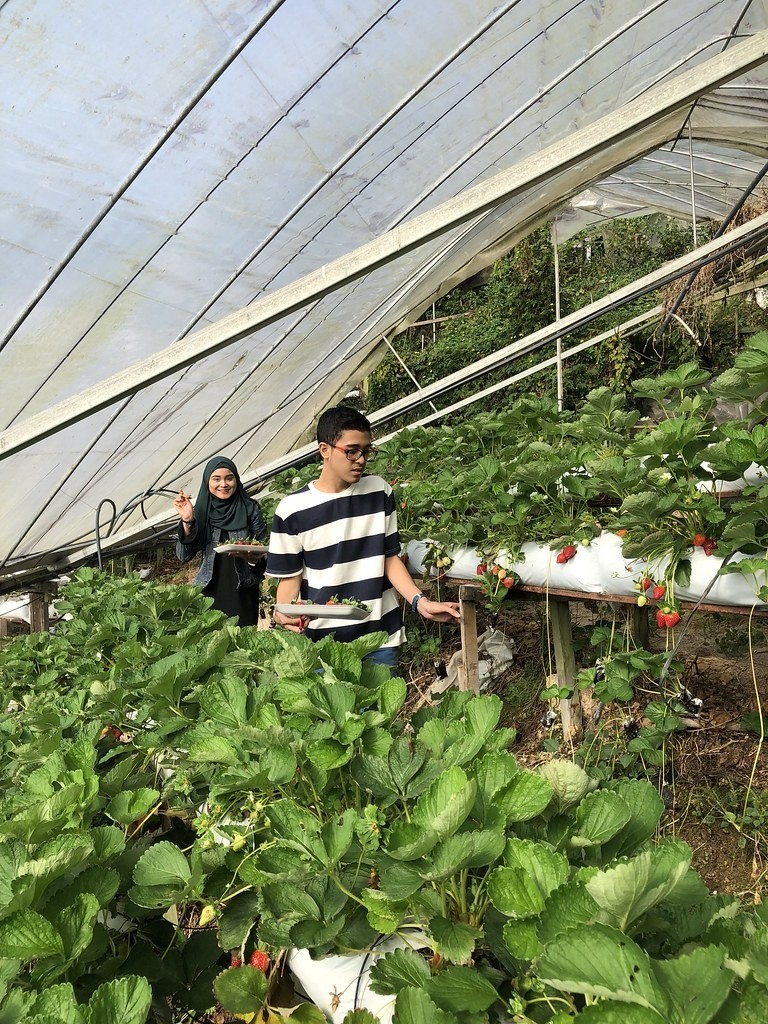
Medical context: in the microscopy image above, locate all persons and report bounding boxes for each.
[264,405,460,714]
[175,456,270,629]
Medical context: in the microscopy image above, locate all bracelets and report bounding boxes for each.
[181,519,194,524]
[412,594,428,614]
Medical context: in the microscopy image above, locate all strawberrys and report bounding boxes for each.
[230,940,270,973]
[102,724,121,739]
[398,530,718,628]
[217,536,264,546]
[293,593,339,605]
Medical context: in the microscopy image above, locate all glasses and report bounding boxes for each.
[325,442,378,462]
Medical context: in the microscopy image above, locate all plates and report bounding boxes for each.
[213,544,270,554]
[276,604,370,620]
